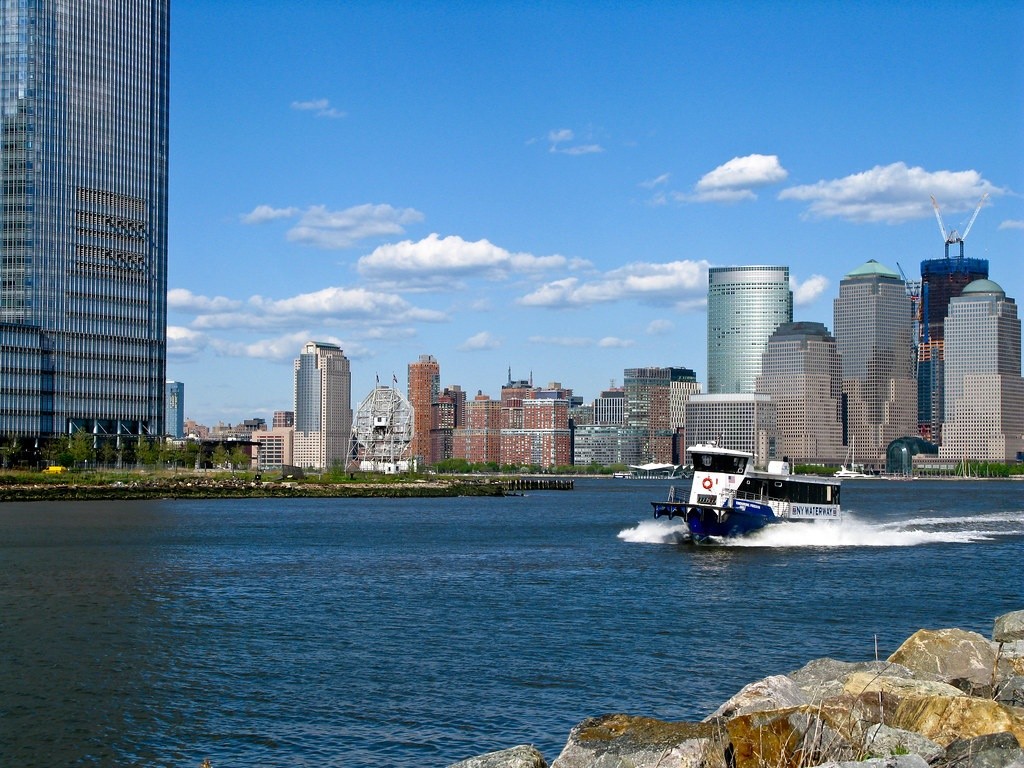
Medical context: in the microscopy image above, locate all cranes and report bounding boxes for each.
[928,190,990,260]
[894,261,921,350]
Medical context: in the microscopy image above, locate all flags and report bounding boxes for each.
[394,375,397,383]
[377,375,379,382]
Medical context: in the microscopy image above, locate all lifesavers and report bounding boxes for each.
[702,478,713,489]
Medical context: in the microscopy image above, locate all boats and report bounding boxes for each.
[650,410,845,544]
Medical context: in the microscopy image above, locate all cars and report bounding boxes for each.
[41,465,68,475]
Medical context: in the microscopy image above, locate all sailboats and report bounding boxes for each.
[833,434,875,478]
